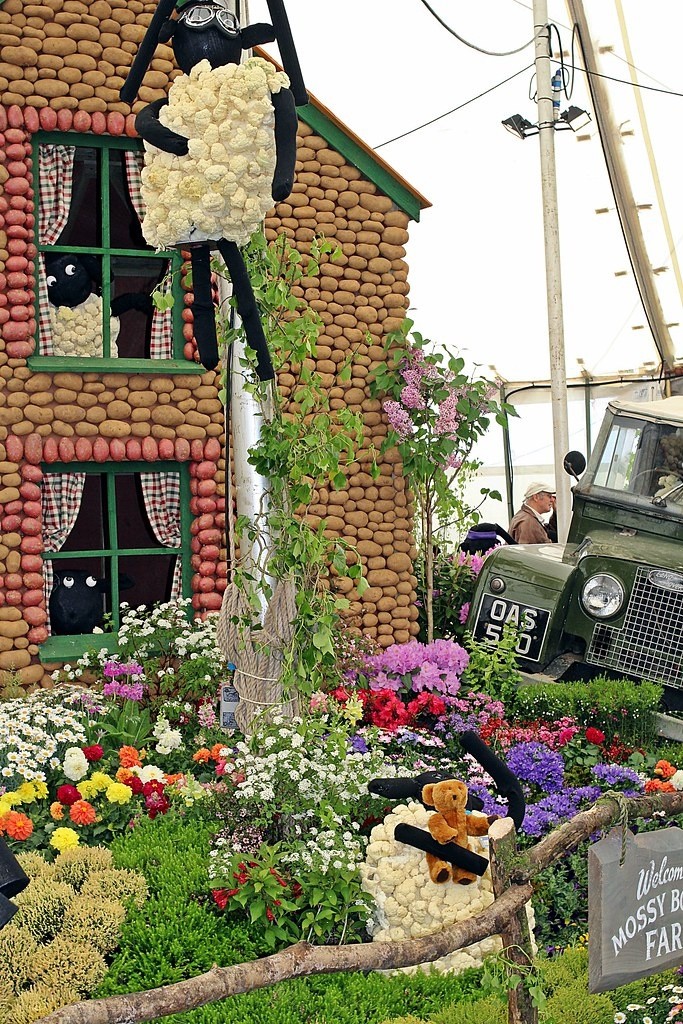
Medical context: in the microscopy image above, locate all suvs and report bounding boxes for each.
[465,397,683,744]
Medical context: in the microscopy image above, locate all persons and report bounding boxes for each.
[457,522,519,557]
[508,482,558,545]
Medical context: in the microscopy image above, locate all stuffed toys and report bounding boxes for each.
[421,778,498,885]
[360,730,539,977]
[48,569,135,637]
[120,0,310,382]
[45,252,154,358]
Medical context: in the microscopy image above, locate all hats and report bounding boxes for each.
[523,481,556,502]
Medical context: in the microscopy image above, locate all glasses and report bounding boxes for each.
[540,494,552,499]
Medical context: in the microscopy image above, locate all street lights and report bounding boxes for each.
[502,107,591,546]
